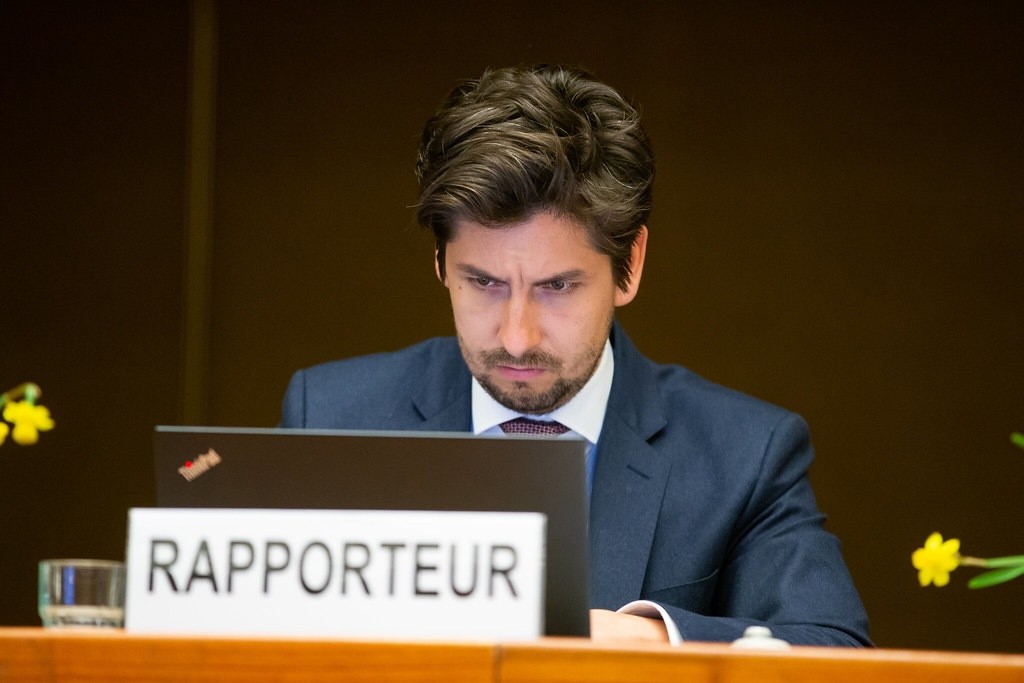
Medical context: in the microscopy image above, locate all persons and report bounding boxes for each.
[276,66,875,650]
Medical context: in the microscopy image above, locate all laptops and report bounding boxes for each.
[154,424,593,642]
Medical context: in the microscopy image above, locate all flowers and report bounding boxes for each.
[0,379,57,451]
[908,436,1024,588]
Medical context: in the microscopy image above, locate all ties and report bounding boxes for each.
[498,417,572,439]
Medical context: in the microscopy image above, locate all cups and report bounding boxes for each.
[37,559,125,628]
